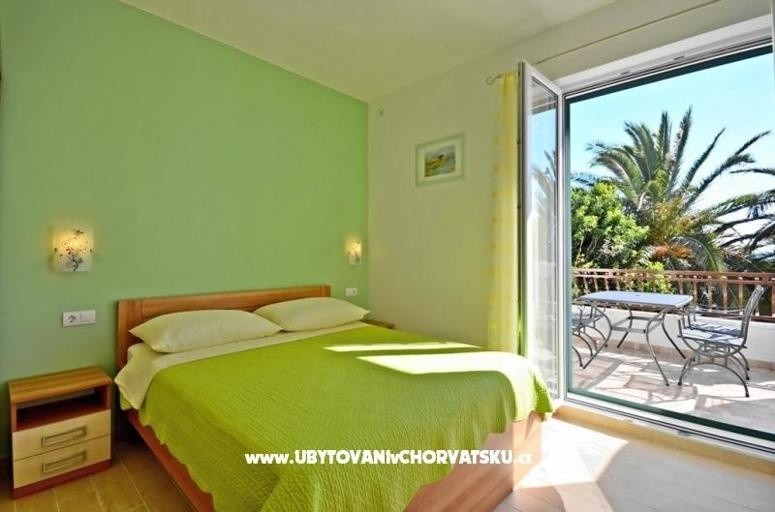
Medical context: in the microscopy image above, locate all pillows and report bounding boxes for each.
[128,310,284,354]
[253,298,372,331]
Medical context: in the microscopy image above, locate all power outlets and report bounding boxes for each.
[63,312,80,326]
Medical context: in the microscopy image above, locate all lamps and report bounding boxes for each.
[53,227,94,271]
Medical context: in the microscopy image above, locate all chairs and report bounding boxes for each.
[570,285,766,399]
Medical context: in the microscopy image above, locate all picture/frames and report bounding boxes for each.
[413,134,466,187]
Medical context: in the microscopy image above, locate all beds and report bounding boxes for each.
[113,285,553,511]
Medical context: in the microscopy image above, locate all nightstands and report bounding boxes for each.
[8,366,115,499]
